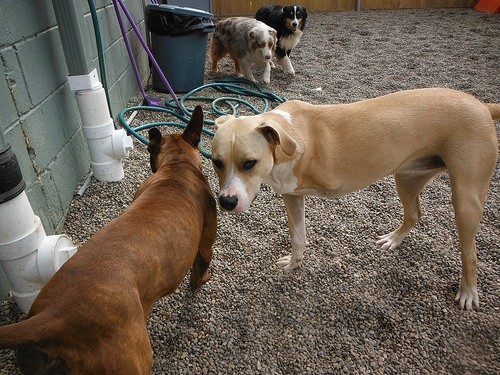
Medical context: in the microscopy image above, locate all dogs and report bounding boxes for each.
[211,16,278,83]
[0,104,218,374]
[212,88,500,310]
[255,4,308,79]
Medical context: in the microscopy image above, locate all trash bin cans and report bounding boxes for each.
[145,3,215,94]
